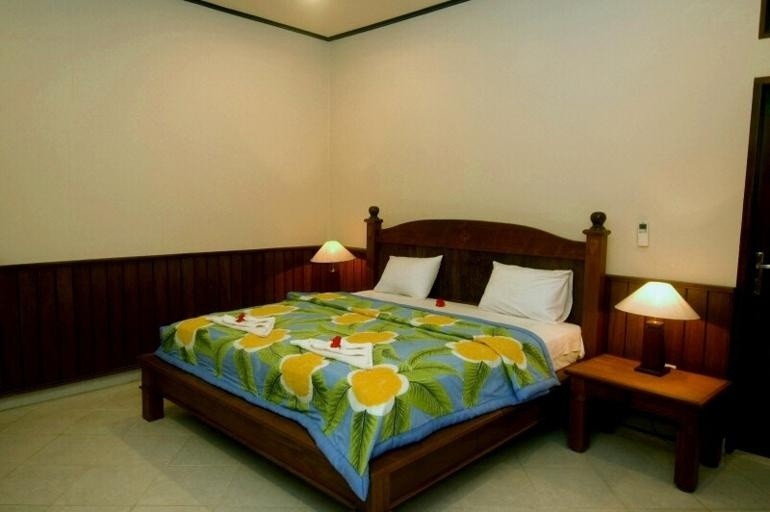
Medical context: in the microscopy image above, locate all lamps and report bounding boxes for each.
[614,281,701,377]
[310,240,356,273]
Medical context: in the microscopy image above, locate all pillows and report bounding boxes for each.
[477,259,576,326]
[374,253,444,301]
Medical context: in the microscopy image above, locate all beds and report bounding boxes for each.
[140,207,612,511]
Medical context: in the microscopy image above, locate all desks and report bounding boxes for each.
[563,352,731,494]
